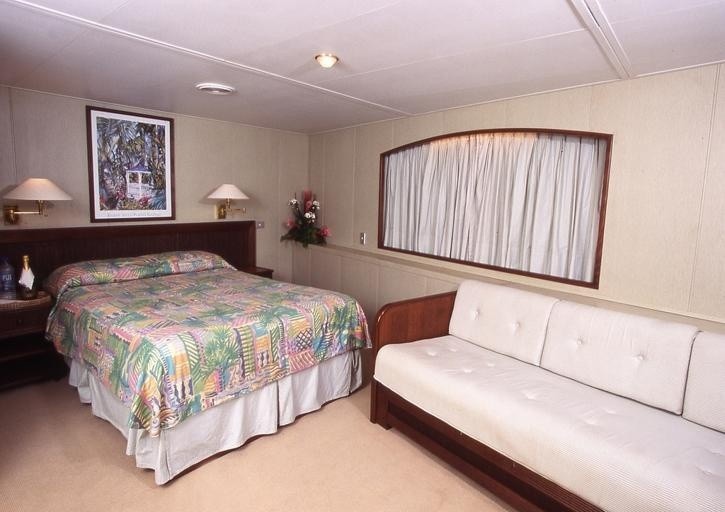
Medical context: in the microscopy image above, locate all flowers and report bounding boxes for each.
[280,190,330,249]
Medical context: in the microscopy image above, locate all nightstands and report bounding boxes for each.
[1,289,63,393]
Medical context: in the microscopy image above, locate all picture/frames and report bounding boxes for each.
[86,106,176,223]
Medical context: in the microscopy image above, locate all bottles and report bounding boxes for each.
[1,254,38,300]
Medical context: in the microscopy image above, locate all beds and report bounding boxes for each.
[1,221,366,484]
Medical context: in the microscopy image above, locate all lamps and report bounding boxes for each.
[315,54,338,69]
[206,183,251,220]
[1,178,73,226]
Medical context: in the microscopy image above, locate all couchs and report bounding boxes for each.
[370,278,724,511]
